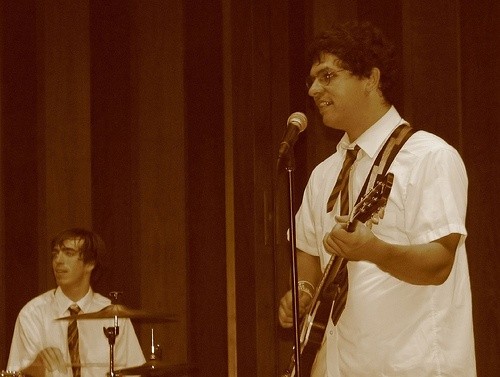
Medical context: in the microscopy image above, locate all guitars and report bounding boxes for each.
[285,173,395,377]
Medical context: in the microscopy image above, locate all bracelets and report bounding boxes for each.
[295,281,316,297]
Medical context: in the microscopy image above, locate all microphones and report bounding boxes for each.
[279,112,307,159]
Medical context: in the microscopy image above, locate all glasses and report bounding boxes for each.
[305,69,345,89]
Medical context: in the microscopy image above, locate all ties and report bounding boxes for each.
[67,305,82,377]
[327,144,361,327]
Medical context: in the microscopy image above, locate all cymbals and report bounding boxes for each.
[107,355,196,376]
[52,302,177,323]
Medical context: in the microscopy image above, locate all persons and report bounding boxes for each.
[6,229,145,377]
[278,22,477,377]
